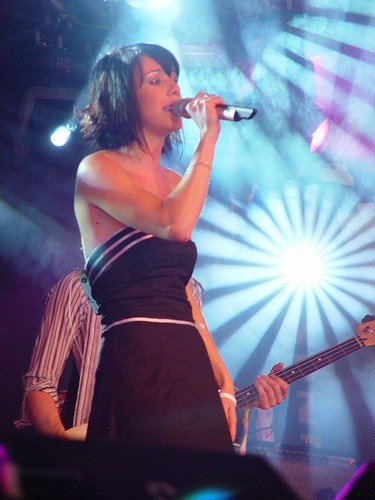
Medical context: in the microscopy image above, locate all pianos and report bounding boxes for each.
[233,436,359,469]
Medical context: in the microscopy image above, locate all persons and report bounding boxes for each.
[71,43,237,455]
[10,265,289,448]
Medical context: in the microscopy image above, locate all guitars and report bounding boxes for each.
[236,315,375,408]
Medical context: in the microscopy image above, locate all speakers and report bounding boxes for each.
[334,459,375,500]
[0,426,302,500]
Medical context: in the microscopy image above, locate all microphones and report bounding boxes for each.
[173,98,257,121]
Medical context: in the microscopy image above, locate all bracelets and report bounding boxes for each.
[184,163,215,171]
[219,391,238,403]
